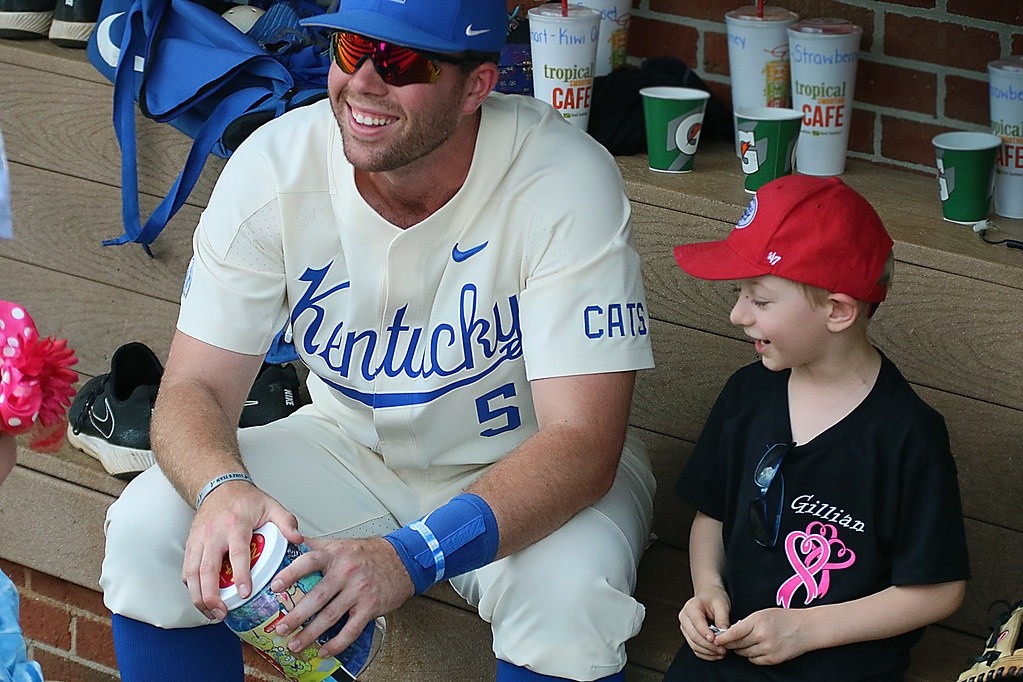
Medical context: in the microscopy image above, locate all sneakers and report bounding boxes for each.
[0,0,54,39]
[64,340,166,480]
[48,0,103,47]
[236,365,300,427]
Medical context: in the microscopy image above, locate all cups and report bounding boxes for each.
[568,0,631,79]
[724,6,801,158]
[640,88,710,173]
[932,132,1003,225]
[734,108,805,194]
[527,3,602,132]
[787,19,863,176]
[988,58,1023,219]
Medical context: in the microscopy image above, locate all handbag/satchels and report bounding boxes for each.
[86,0,332,260]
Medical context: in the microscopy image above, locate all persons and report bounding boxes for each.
[98,0,657,682]
[660,177,973,682]
[0,299,79,682]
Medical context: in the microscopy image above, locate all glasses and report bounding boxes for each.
[328,30,464,88]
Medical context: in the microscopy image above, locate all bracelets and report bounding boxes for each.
[195,473,254,510]
[382,492,500,594]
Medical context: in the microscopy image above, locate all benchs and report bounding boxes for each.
[0,40,1023,682]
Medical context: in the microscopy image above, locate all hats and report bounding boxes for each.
[299,0,507,60]
[672,174,887,304]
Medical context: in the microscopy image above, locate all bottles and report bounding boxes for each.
[218,522,386,682]
